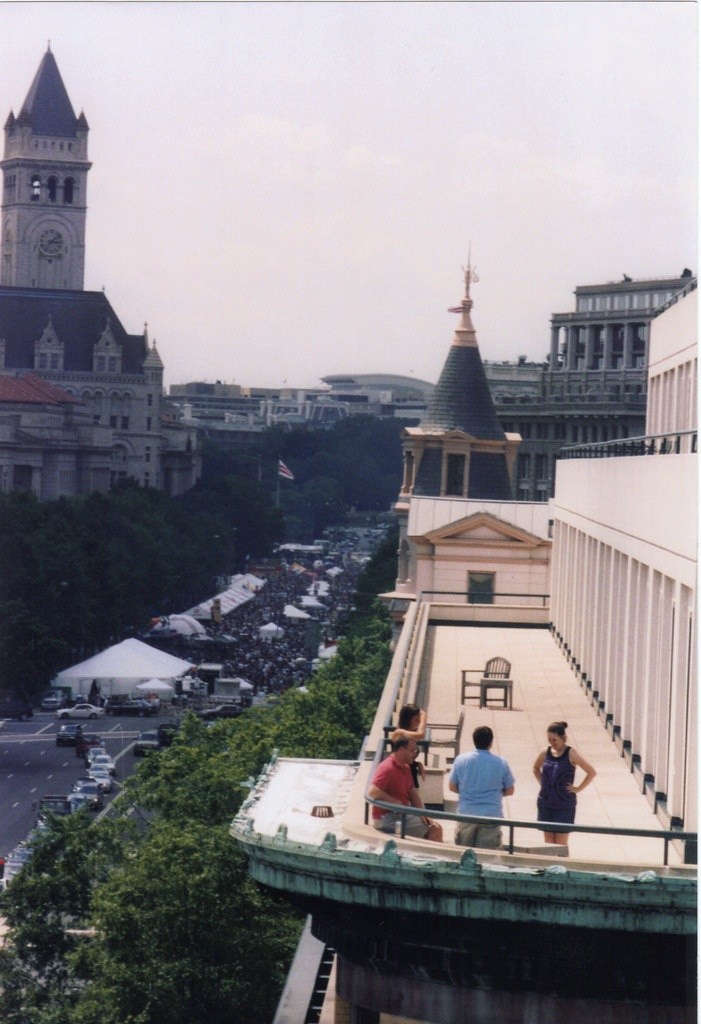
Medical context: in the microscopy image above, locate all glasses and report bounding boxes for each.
[403,746,419,755]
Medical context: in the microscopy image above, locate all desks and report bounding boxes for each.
[480,678,513,710]
[383,726,430,766]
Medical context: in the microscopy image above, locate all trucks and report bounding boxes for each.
[37,795,72,831]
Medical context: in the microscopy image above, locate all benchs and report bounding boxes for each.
[502,842,568,858]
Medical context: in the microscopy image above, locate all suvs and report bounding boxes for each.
[75,734,105,758]
[56,723,82,746]
[76,783,104,811]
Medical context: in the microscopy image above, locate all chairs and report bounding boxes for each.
[425,712,464,760]
[461,656,511,707]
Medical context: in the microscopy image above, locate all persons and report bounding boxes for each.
[448,726,516,848]
[532,721,597,858]
[367,738,444,843]
[391,703,425,788]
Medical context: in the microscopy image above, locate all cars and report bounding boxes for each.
[0,701,33,720]
[1,819,62,897]
[196,704,247,722]
[68,793,88,816]
[133,731,158,755]
[314,522,391,553]
[86,771,112,792]
[86,764,111,773]
[55,703,105,719]
[88,755,115,774]
[71,777,96,795]
[85,748,108,767]
[105,699,158,717]
[156,723,178,746]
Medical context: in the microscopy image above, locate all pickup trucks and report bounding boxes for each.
[41,690,67,710]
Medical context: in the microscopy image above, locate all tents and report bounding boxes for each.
[268,604,317,623]
[143,573,265,637]
[50,638,196,699]
[258,622,285,643]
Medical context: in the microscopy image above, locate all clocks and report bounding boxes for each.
[37,228,65,256]
